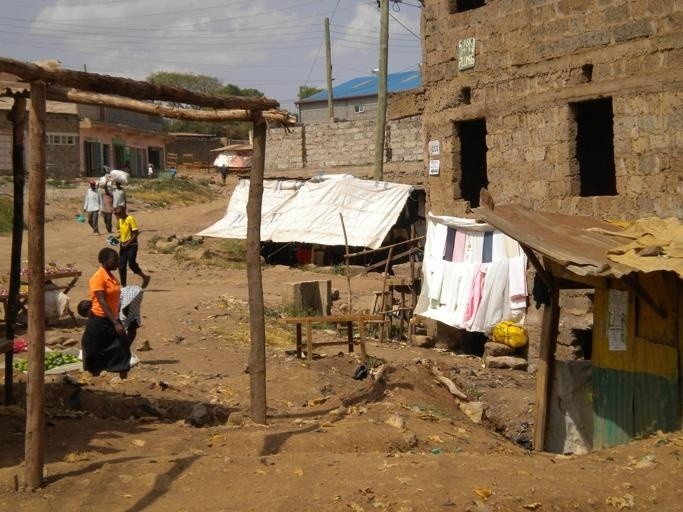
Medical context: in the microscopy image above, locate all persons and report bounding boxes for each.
[219,165,228,185]
[82,249,130,380]
[146,160,154,178]
[122,160,130,172]
[259,240,279,264]
[83,174,128,238]
[115,205,150,288]
[78,285,143,346]
[167,166,177,180]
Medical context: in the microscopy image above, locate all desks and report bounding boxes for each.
[0,294,29,323]
[20,270,82,329]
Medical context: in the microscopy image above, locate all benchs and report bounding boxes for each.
[277,314,384,363]
